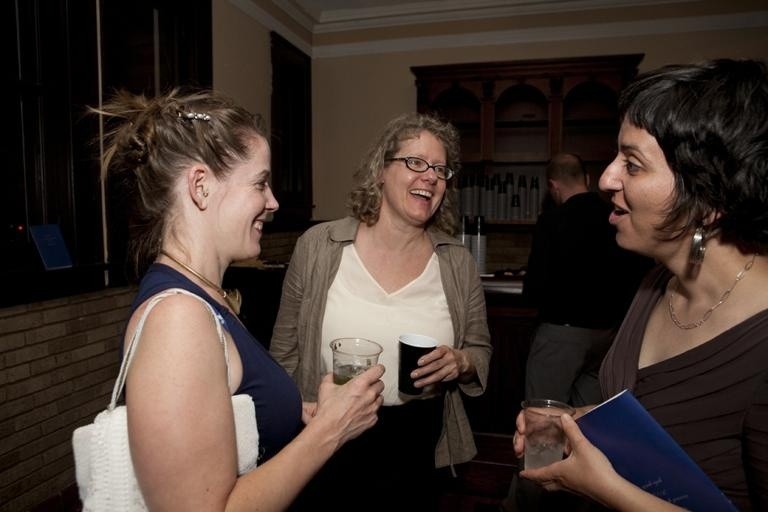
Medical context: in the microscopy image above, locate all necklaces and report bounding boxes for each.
[668,254,756,329]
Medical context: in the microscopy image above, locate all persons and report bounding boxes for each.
[523,152,637,408]
[88,88,386,512]
[514,59,767,511]
[268,113,493,512]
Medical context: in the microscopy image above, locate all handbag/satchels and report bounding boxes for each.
[69,391,260,512]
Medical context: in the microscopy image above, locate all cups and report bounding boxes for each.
[521,398,576,470]
[452,214,490,277]
[329,338,384,385]
[454,172,539,221]
[397,334,438,395]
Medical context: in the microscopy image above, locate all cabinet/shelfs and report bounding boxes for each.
[408,51,650,319]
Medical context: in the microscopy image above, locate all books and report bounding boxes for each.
[573,390,739,512]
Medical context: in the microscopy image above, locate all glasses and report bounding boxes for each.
[386,155,453,182]
